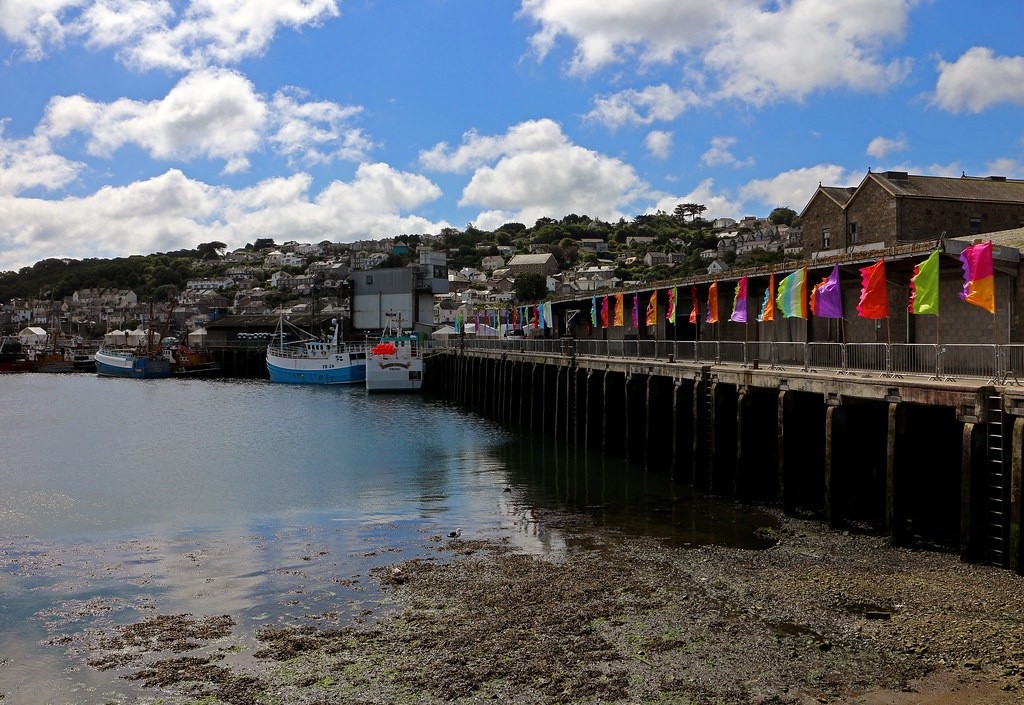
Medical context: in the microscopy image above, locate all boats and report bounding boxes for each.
[0,324,98,375]
[177,361,224,381]
[364,308,427,393]
[265,303,366,386]
[95,295,178,380]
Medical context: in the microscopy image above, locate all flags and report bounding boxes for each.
[538,304,544,329]
[960,242,995,313]
[533,305,538,328]
[647,291,657,326]
[475,306,528,332]
[810,264,842,318]
[590,297,597,327]
[731,276,747,322]
[910,250,939,315]
[543,301,552,328]
[776,267,807,320]
[857,259,889,319]
[633,296,638,328]
[601,297,608,328]
[756,274,774,322]
[613,292,623,326]
[706,283,719,323]
[689,288,698,323]
[666,287,677,323]
